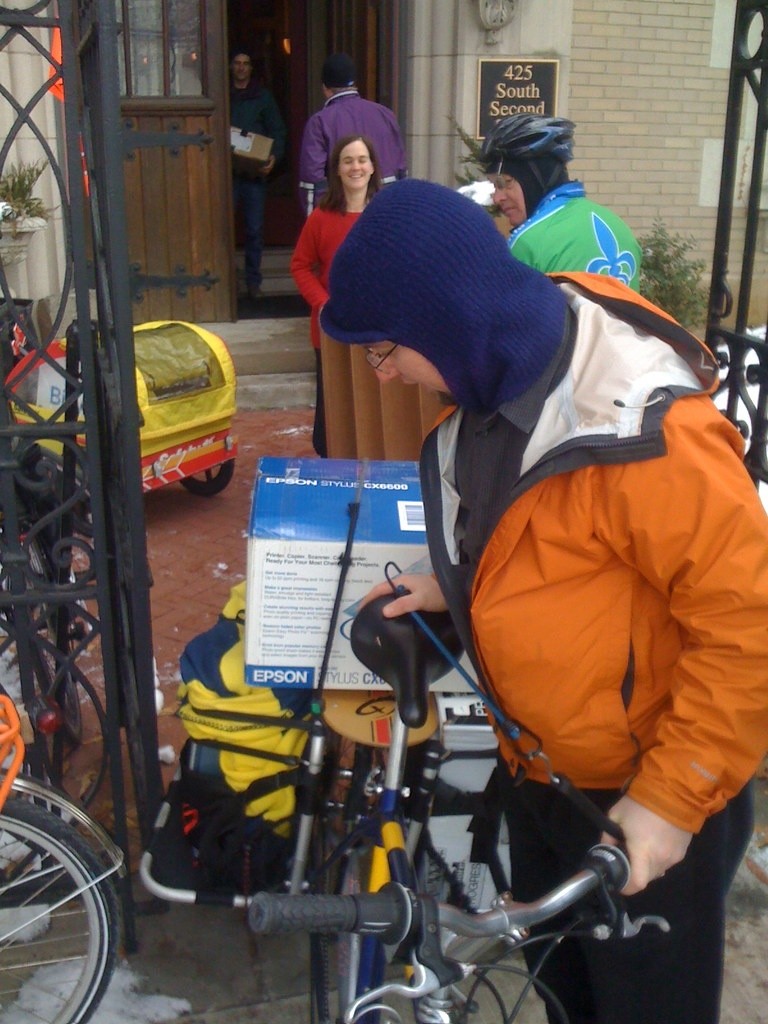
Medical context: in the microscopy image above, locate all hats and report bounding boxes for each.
[319,179,567,420]
[320,51,358,87]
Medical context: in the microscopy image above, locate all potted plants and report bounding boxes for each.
[0,156,62,299]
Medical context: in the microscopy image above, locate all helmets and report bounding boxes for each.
[482,113,575,193]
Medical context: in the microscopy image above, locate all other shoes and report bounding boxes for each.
[249,286,267,302]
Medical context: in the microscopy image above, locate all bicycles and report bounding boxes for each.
[248,588,671,1024]
[0,516,128,1024]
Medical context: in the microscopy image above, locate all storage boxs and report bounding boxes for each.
[243,457,479,693]
[231,126,275,175]
[415,686,511,944]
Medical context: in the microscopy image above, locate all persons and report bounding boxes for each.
[298,56,406,223]
[320,178,768,1024]
[292,138,379,456]
[228,42,287,298]
[480,113,642,296]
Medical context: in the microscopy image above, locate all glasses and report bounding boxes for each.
[367,344,397,372]
